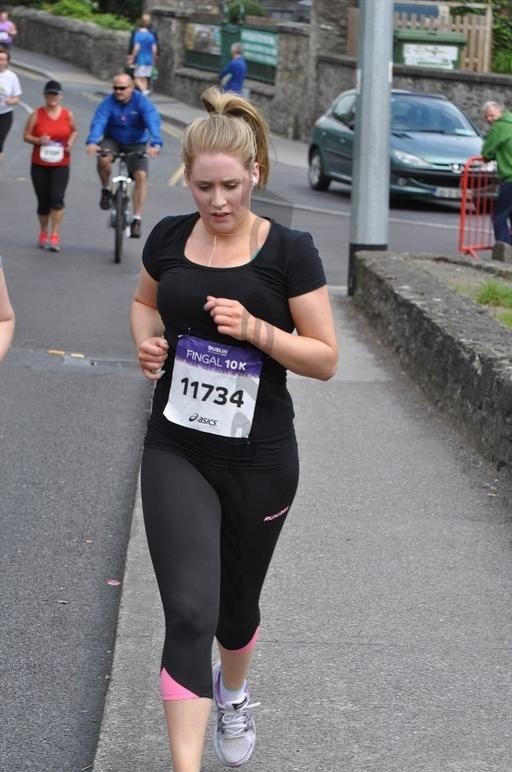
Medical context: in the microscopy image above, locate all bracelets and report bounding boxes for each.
[152,144,162,148]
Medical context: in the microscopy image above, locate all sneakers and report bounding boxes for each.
[212,662,262,767]
[39,231,60,252]
[131,219,140,237]
[101,189,111,209]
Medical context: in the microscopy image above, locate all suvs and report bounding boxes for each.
[308,89,501,213]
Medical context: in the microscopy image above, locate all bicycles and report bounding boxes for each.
[96,145,149,263]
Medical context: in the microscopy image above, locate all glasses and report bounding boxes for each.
[114,87,125,90]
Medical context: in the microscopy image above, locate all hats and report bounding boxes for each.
[45,81,61,94]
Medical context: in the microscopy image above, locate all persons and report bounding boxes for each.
[125,12,160,97]
[217,42,248,93]
[480,98,511,245]
[128,87,342,772]
[121,16,160,90]
[21,78,79,252]
[0,2,19,62]
[0,45,23,163]
[0,259,18,370]
[83,73,165,240]
